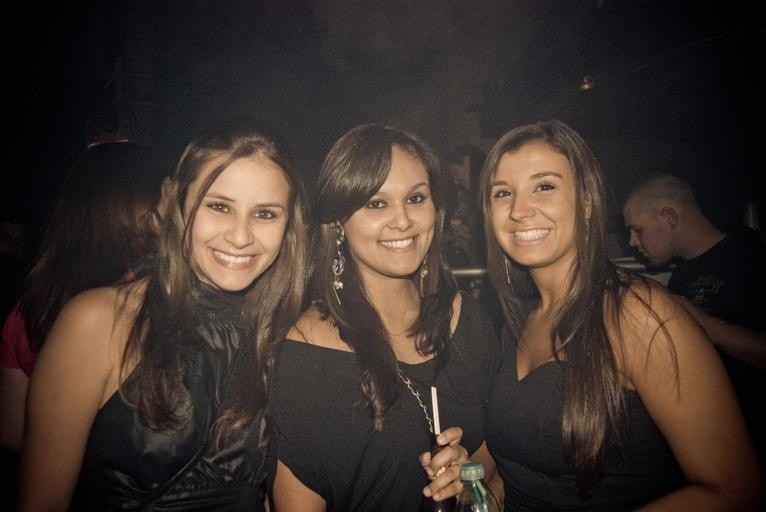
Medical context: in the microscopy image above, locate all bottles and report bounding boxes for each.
[454,463,501,512]
[421,434,458,512]
